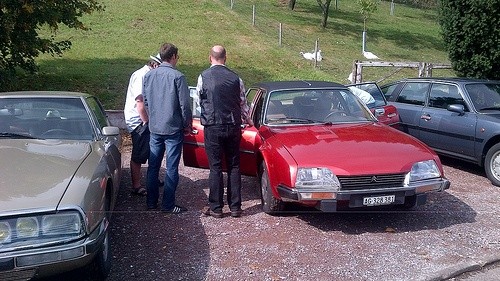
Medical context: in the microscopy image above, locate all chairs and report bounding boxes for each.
[0,113,24,133]
[40,109,73,135]
[266,100,283,115]
[293,96,312,120]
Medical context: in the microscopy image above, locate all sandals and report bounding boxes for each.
[131,186,147,196]
[158,178,164,186]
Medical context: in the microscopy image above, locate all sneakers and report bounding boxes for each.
[163,203,188,214]
[148,201,158,209]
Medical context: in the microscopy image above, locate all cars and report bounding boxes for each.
[364,78,500,187]
[182,80,451,216]
[0,91,123,274]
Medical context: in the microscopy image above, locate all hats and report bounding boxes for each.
[150,53,162,64]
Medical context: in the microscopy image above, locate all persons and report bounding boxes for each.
[196,46,250,218]
[143,44,192,214]
[124,52,164,196]
[329,86,375,112]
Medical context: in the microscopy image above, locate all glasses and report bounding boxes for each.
[176,54,180,59]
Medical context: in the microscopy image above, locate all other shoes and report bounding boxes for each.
[202,207,223,218]
[230,208,242,217]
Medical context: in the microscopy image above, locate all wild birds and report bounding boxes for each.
[362,50,381,60]
[347,72,353,83]
[299,50,323,62]
